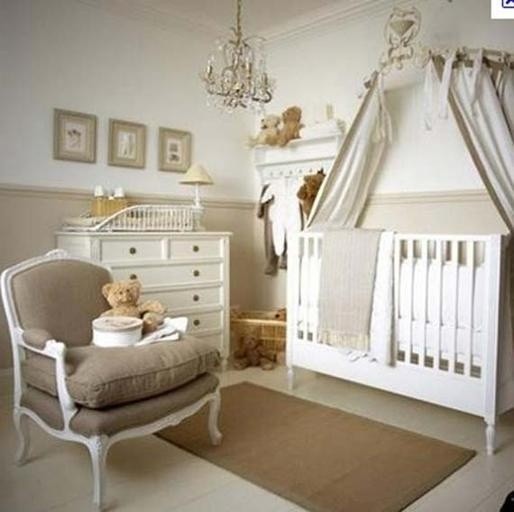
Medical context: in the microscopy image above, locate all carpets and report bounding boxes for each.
[154,380,481,512]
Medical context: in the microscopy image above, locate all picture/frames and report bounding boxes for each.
[51,108,194,176]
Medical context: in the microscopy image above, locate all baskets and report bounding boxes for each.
[231,304,287,351]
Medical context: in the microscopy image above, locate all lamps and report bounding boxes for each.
[199,0,274,117]
[178,159,215,231]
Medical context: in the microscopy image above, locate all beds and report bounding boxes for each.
[285,226,514,456]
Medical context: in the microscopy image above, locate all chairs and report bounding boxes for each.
[1,248,226,512]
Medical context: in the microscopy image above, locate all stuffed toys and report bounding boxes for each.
[272,306,286,321]
[263,105,304,147]
[98,279,167,332]
[231,329,283,371]
[239,114,280,147]
[295,171,325,218]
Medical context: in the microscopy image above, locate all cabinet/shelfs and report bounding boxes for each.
[55,229,233,377]
